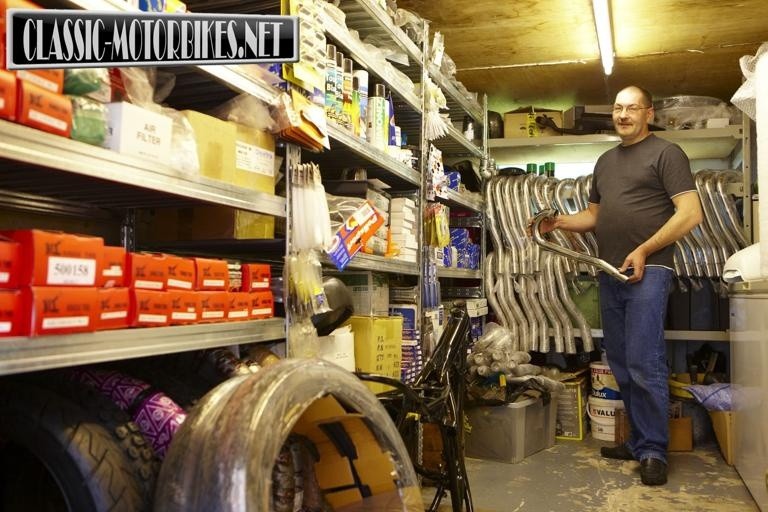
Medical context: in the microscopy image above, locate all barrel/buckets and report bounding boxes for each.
[585,395,626,443]
[588,361,624,400]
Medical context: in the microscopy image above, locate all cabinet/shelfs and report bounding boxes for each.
[487,125,751,342]
[0,0,489,433]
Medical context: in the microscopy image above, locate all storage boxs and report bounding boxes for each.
[707,411,732,466]
[503,105,564,139]
[465,389,558,463]
[556,367,589,440]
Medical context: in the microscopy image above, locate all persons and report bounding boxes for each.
[526,84,704,485]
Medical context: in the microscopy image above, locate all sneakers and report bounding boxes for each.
[642,459,667,485]
[601,446,636,460]
